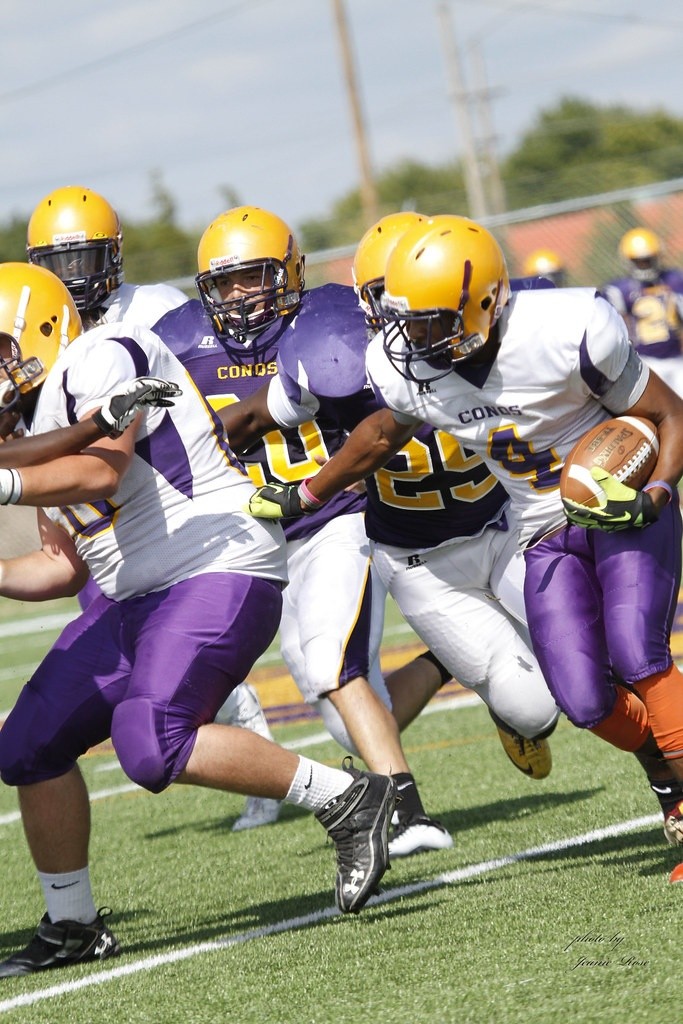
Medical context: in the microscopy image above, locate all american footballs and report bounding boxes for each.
[561,415,660,512]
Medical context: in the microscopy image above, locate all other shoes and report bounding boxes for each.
[662,798,683,885]
[235,799,281,828]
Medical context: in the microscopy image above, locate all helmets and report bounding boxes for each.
[620,228,662,262]
[195,207,306,343]
[0,261,85,415]
[29,186,121,314]
[519,250,570,278]
[351,211,512,385]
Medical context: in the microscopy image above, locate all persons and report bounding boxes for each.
[0,261,407,980]
[25,188,681,883]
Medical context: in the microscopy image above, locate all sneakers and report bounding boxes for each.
[314,756,403,915]
[488,707,553,781]
[0,906,122,979]
[389,816,453,858]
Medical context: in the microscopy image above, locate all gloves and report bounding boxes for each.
[561,466,659,537]
[92,377,182,441]
[246,481,313,520]
